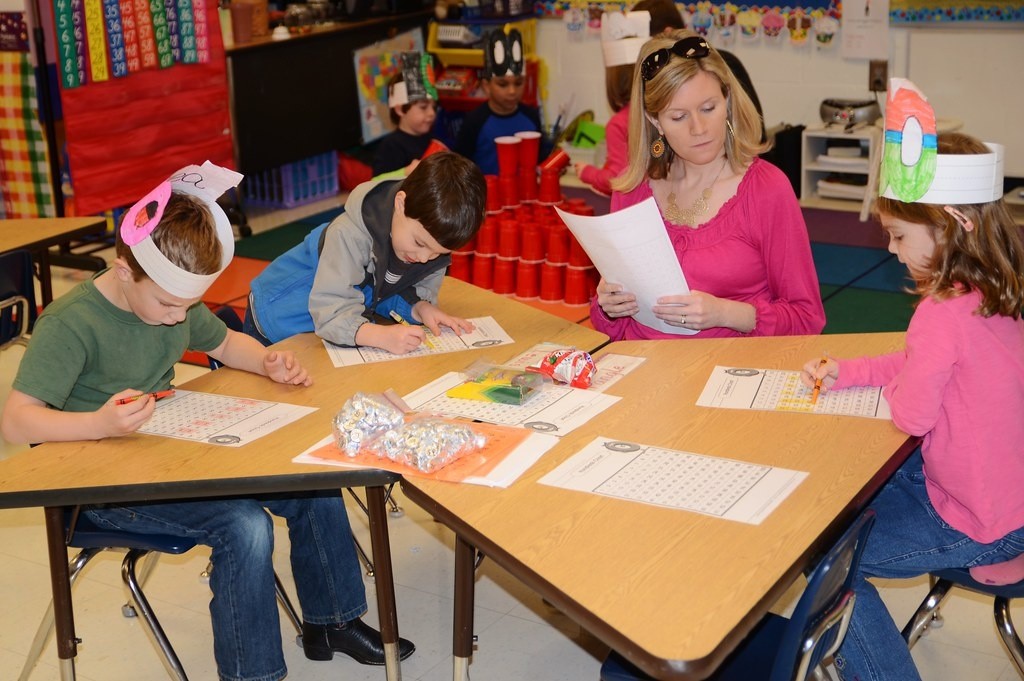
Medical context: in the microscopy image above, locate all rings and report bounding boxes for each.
[682,315,686,325]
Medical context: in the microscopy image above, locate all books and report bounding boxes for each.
[818,173,868,199]
[817,140,869,163]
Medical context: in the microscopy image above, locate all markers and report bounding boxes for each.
[389,310,435,350]
[811,355,828,404]
[116,390,176,406]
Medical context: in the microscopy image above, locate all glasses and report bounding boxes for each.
[641,36,711,90]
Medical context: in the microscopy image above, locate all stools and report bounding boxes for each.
[899,570,1024,677]
[59,497,306,681]
[209,306,405,577]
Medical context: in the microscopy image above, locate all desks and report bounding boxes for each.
[1,216,926,681]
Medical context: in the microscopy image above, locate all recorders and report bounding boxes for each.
[820,98,880,125]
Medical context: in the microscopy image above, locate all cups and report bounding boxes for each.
[449,131,601,310]
[419,140,449,161]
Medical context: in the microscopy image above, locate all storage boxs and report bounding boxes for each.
[239,151,338,209]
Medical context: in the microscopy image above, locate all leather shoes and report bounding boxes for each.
[302,617,415,665]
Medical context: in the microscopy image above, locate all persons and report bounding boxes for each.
[372,53,446,180]
[589,28,827,342]
[459,28,553,173]
[808,76,1024,681]
[1,160,417,680]
[208,152,487,370]
[573,0,685,193]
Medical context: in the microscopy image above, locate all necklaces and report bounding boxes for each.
[666,160,728,225]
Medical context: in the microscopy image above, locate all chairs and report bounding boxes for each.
[599,511,876,681]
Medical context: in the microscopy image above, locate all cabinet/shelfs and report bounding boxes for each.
[800,123,882,204]
